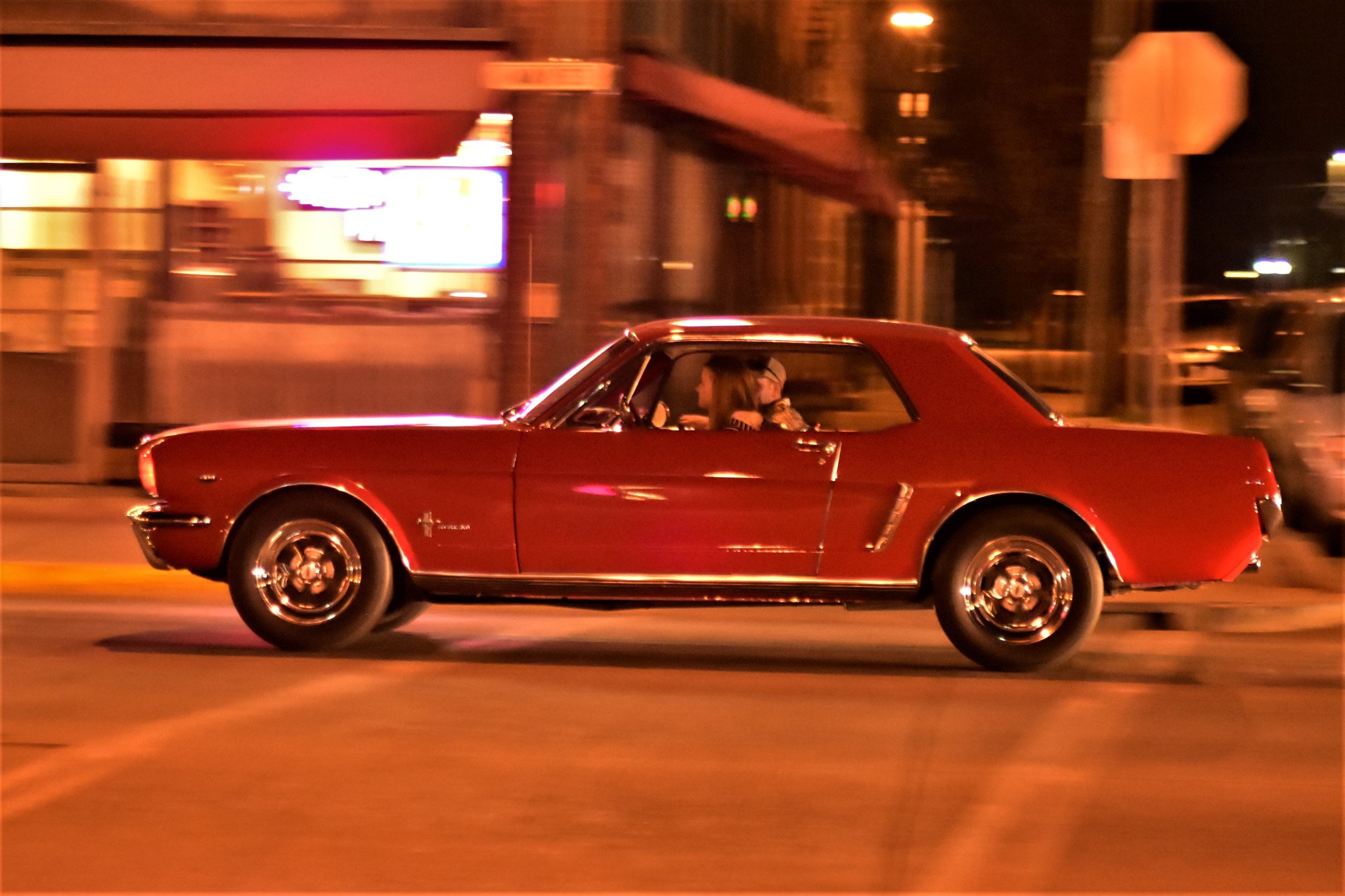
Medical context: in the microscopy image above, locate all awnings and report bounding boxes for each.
[622,57,902,221]
[0,47,512,158]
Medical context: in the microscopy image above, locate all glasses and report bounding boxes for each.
[747,359,782,383]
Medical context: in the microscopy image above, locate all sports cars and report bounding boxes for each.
[124,311,1285,676]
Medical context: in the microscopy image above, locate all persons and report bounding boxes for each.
[678,355,806,432]
[696,356,764,432]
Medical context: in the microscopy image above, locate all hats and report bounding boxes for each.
[744,354,786,388]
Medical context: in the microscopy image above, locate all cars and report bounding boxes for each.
[1161,284,1345,555]
